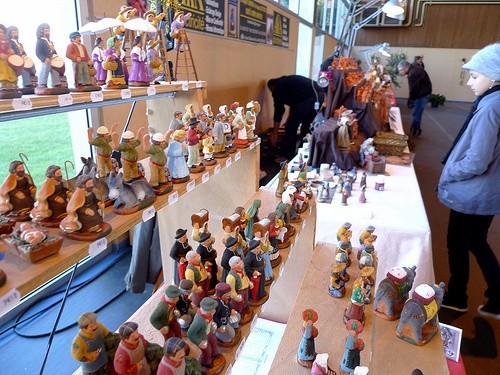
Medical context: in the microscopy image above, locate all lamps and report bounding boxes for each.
[348,0,408,57]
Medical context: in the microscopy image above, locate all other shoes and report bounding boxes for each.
[477,302,500,320]
[438,294,469,322]
[276,157,287,163]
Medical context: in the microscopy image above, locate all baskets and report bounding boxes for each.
[373,130,409,155]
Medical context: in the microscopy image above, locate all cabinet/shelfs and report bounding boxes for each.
[0,81,449,375]
[307,67,391,171]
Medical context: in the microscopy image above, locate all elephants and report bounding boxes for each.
[374,265,445,340]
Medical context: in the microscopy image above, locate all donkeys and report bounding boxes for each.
[109,171,155,208]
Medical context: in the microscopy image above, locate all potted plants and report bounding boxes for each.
[430,94,445,108]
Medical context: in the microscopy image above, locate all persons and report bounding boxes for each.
[397,60,432,138]
[434,42,500,319]
[297,222,378,375]
[407,56,425,109]
[267,74,325,161]
[275,105,368,207]
[70,160,327,375]
[0,5,194,103]
[0,100,261,289]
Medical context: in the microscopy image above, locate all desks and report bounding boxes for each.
[265,107,436,299]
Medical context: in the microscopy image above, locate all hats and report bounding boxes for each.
[461,42,500,81]
